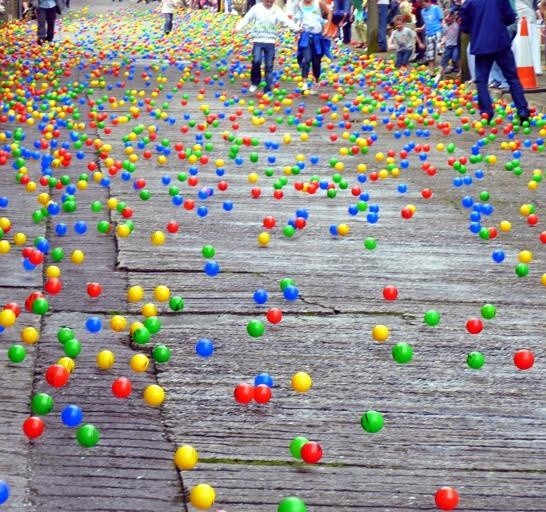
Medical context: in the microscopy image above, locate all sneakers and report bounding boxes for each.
[487,110,496,125]
[425,63,464,84]
[408,53,433,66]
[519,116,531,127]
[301,82,319,95]
[249,85,258,93]
[334,35,368,49]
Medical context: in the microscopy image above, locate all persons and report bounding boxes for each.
[21,0,70,45]
[186,1,218,13]
[154,0,179,35]
[228,1,366,95]
[377,0,546,125]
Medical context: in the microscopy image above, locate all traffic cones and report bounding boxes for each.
[501,15,546,93]
